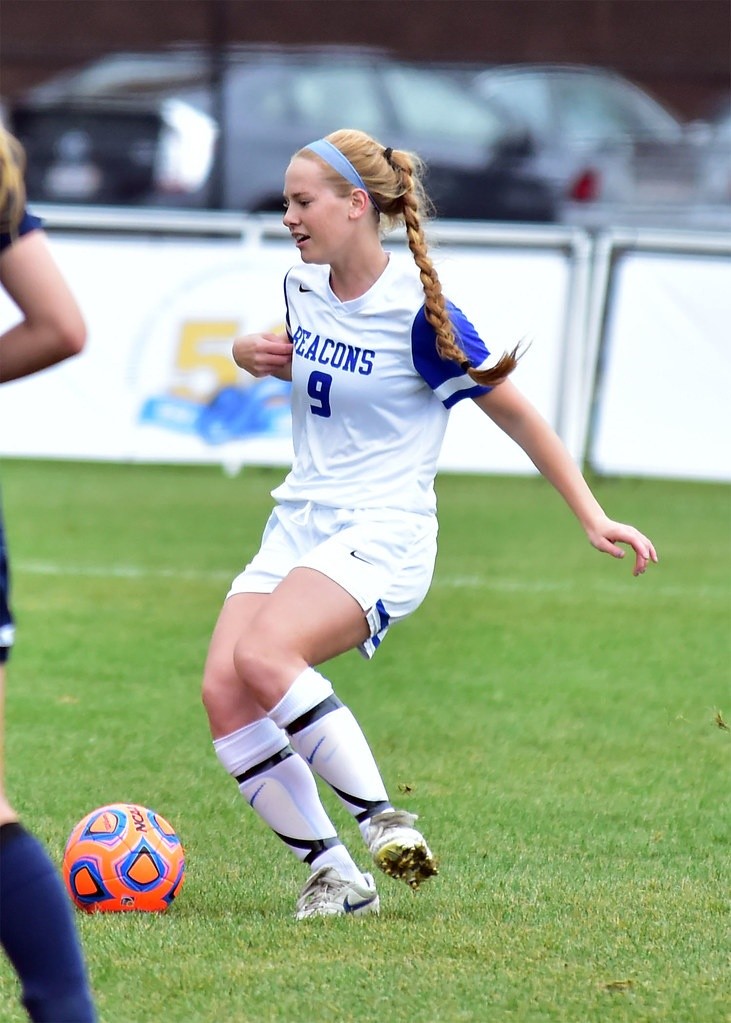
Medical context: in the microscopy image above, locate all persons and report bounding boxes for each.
[200,128,659,923]
[0,117,100,1022]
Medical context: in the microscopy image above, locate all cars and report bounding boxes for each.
[17,46,729,229]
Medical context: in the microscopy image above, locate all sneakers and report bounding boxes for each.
[368,810,440,892]
[293,864,380,922]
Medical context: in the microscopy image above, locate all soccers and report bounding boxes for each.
[59,803,188,914]
